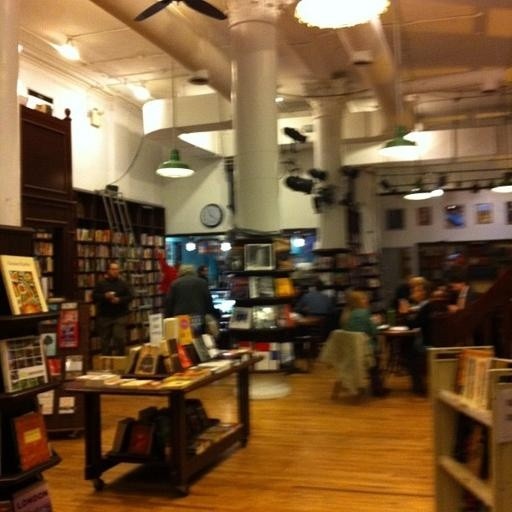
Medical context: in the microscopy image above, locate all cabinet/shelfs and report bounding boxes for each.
[426,346,512,512]
[21,193,78,299]
[315,248,383,315]
[0,311,61,484]
[76,218,164,356]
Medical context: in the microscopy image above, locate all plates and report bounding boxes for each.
[389,326,409,331]
[377,324,389,330]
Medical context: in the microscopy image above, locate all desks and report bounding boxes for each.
[377,326,421,378]
[63,351,265,497]
[227,316,324,373]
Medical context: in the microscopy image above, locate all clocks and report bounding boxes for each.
[200,203,223,228]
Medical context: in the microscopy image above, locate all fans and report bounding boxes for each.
[132,0,229,23]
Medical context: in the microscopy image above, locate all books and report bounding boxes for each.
[37,225,257,456]
[453,349,512,511]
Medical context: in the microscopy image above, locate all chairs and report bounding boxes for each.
[331,329,372,405]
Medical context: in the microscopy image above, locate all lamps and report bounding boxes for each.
[292,0,390,30]
[375,164,512,201]
[384,0,416,148]
[155,59,195,179]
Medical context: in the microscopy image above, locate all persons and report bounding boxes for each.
[340,274,486,398]
[292,279,330,356]
